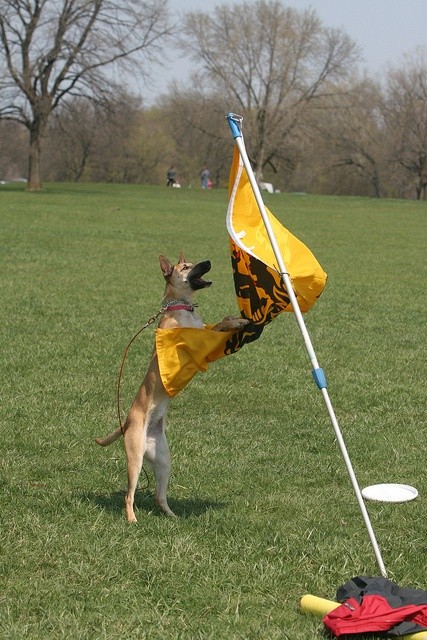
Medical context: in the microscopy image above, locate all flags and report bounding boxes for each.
[152,112,327,397]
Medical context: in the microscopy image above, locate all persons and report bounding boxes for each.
[166,166,176,187]
[199,165,209,189]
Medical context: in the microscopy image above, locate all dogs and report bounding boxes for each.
[94,251,250,523]
